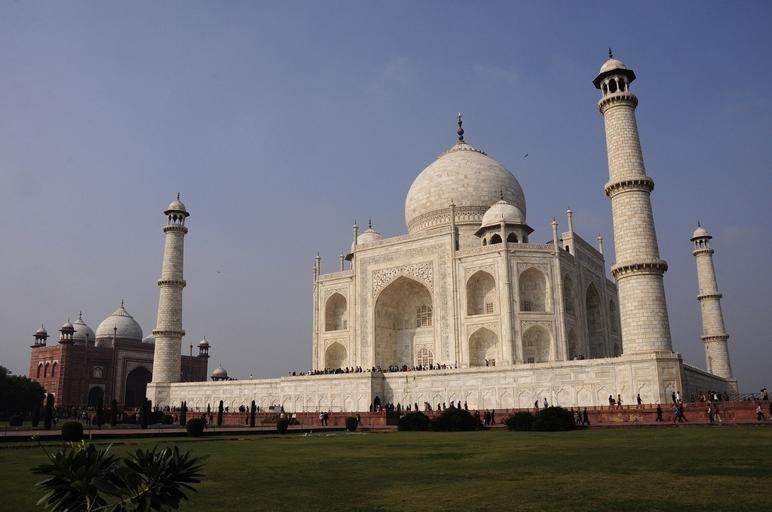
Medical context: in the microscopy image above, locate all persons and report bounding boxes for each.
[290,360,459,377]
